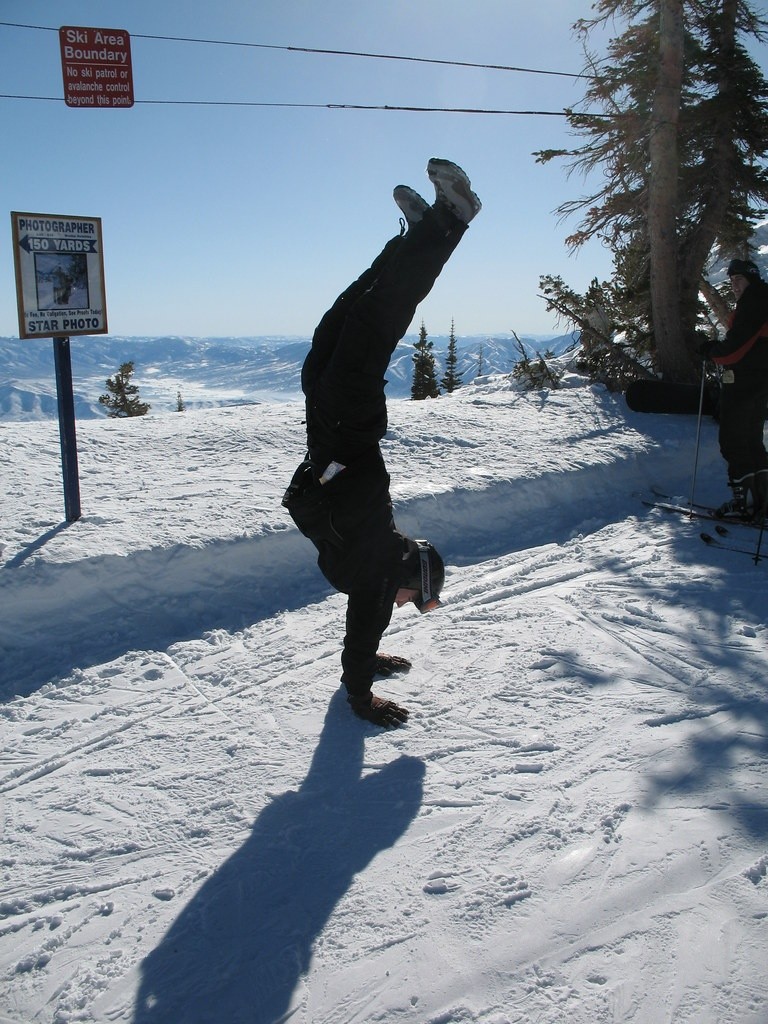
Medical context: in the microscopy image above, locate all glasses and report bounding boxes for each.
[415,540,443,614]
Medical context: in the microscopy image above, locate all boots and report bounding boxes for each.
[747,470,768,528]
[716,473,755,524]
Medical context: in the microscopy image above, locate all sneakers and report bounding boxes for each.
[425,158,482,225]
[393,185,432,239]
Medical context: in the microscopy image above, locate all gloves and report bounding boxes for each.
[347,691,409,731]
[376,653,412,677]
[695,340,721,361]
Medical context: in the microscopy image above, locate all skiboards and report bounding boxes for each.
[700,525,768,557]
[642,485,768,531]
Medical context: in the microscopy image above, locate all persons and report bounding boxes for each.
[284,158,480,729]
[694,259,767,522]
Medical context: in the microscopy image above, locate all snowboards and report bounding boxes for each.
[626,379,721,413]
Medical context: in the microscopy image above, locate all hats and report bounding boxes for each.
[727,260,761,284]
[400,537,445,610]
